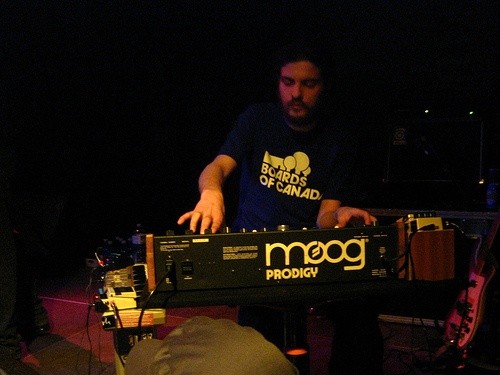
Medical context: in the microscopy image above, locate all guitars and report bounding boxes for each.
[446,206,500,348]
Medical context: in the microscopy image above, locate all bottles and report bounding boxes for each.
[132,225,146,262]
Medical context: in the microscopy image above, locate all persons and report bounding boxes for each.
[176,43,378,375]
[122,315,297,375]
